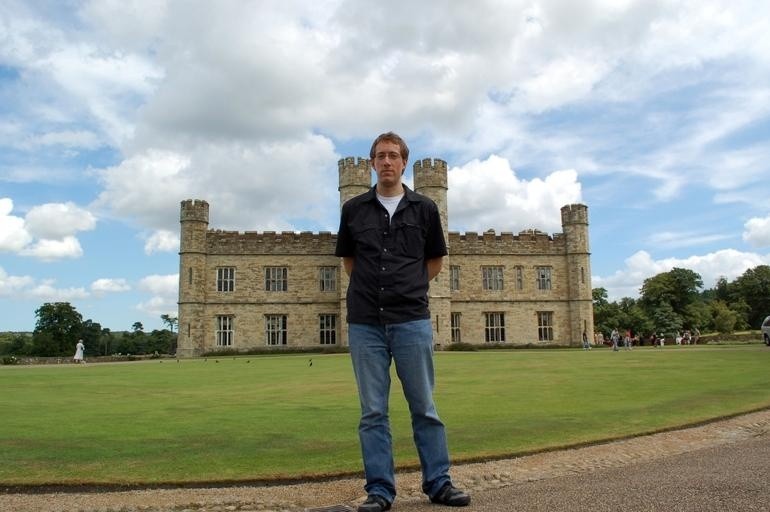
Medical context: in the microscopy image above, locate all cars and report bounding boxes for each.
[760,315,770,346]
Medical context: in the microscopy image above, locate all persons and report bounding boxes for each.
[74,339,85,363]
[583,327,700,351]
[335,132,471,512]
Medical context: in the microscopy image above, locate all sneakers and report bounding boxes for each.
[431,482,470,506]
[358,494,390,512]
